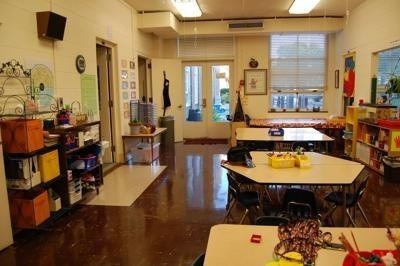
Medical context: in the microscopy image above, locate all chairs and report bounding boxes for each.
[188,117,400,266]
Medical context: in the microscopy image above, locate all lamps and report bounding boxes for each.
[288,0,320,15]
[169,0,203,17]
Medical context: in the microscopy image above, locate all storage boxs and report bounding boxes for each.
[0,117,82,229]
[129,142,160,165]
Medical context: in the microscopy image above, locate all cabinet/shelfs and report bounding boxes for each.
[344,106,368,160]
[0,58,69,236]
[43,119,105,202]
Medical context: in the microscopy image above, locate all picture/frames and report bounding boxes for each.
[335,69,340,88]
[244,68,268,96]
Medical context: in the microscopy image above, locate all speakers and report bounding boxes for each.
[36,11,67,41]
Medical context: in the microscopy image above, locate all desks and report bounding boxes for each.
[120,125,167,167]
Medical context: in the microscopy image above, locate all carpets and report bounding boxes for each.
[76,164,168,208]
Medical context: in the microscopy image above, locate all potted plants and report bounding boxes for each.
[384,76,400,94]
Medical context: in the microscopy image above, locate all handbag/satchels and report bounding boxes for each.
[227,146,252,166]
